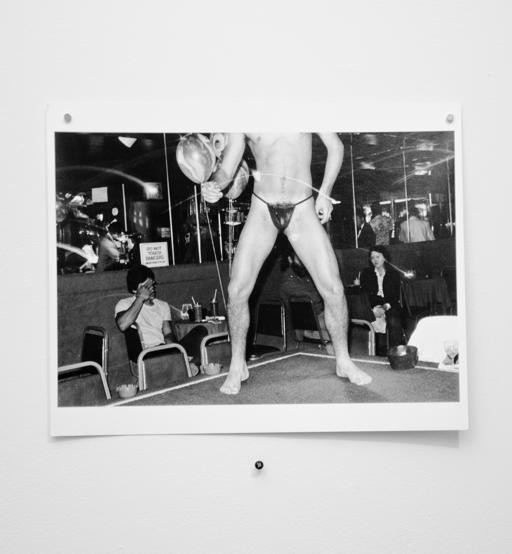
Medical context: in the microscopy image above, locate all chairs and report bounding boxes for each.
[58,266,456,406]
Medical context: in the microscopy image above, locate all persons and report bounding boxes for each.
[398,207,436,242]
[368,202,390,245]
[274,249,331,343]
[359,243,403,348]
[198,132,372,394]
[347,211,377,246]
[114,264,209,376]
[95,221,131,271]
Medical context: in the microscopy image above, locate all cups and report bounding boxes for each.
[193,305,202,323]
[210,300,218,316]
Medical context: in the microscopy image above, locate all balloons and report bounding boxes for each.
[175,132,217,184]
[220,157,251,201]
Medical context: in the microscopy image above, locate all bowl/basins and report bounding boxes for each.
[202,363,223,375]
[387,345,419,370]
[116,384,138,399]
